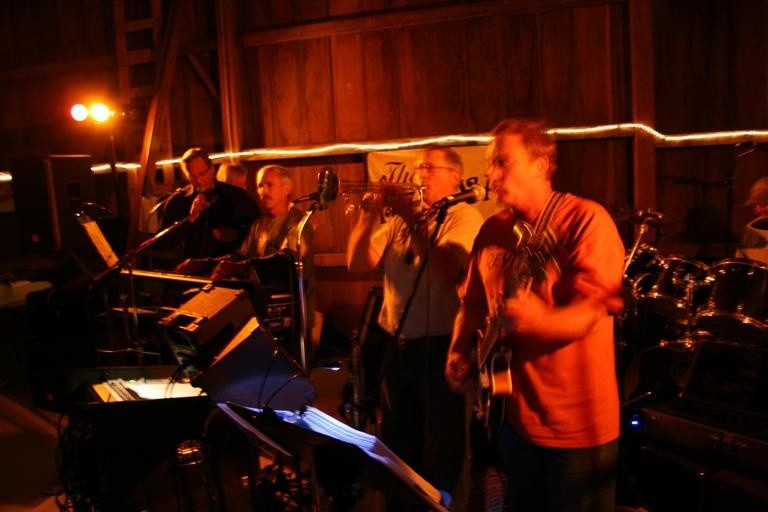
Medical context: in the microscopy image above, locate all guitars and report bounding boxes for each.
[465,226,558,437]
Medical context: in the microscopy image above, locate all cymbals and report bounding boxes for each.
[612,207,674,225]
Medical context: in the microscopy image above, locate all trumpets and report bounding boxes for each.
[317,169,425,205]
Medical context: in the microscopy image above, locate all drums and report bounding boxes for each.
[621,244,663,302]
[648,258,705,318]
[691,257,767,345]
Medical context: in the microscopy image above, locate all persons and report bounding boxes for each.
[345,146,486,510]
[734,176,768,264]
[153,147,262,278]
[443,117,626,511]
[172,164,328,347]
[217,162,248,191]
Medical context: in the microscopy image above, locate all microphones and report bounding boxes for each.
[197,186,205,211]
[433,184,486,209]
[292,189,335,203]
